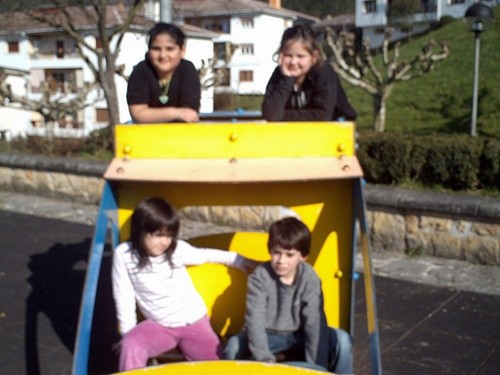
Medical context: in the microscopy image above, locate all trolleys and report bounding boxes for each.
[70,120,386,375]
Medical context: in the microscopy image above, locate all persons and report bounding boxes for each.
[111,197,260,373]
[222,218,356,375]
[127,22,201,123]
[261,23,357,122]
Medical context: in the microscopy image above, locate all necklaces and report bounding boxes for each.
[154,79,170,104]
